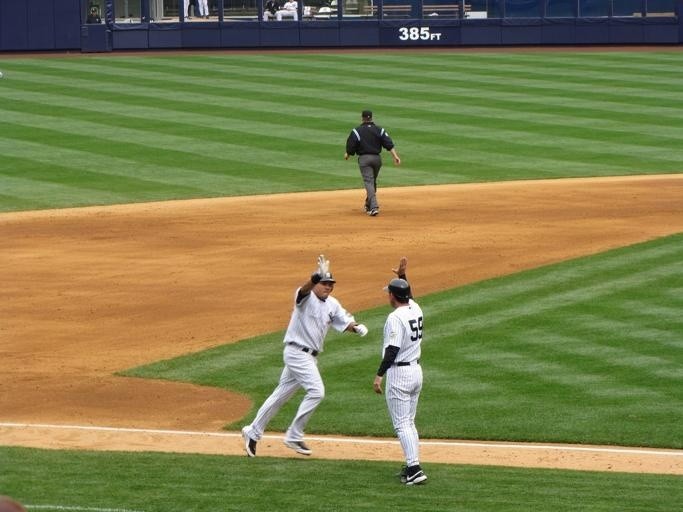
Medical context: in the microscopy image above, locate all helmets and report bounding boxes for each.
[383,278,410,299]
[310,271,336,284]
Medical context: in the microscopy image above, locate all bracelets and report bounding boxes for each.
[398,274,407,282]
[310,273,322,284]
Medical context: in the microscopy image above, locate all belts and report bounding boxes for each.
[289,342,318,357]
[390,360,419,366]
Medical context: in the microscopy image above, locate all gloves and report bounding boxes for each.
[353,324,368,337]
[316,254,330,277]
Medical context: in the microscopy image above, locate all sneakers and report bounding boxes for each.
[242,426,256,457]
[283,437,312,455]
[394,465,427,486]
[364,204,379,216]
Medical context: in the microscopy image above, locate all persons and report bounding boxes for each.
[240,254,368,457]
[86,8,101,24]
[343,110,400,215]
[263,0,280,20]
[183,0,191,22]
[373,257,427,486]
[197,0,208,18]
[276,0,298,20]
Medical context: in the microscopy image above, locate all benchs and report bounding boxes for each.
[264,4,473,20]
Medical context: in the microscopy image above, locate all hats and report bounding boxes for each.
[362,110,372,118]
